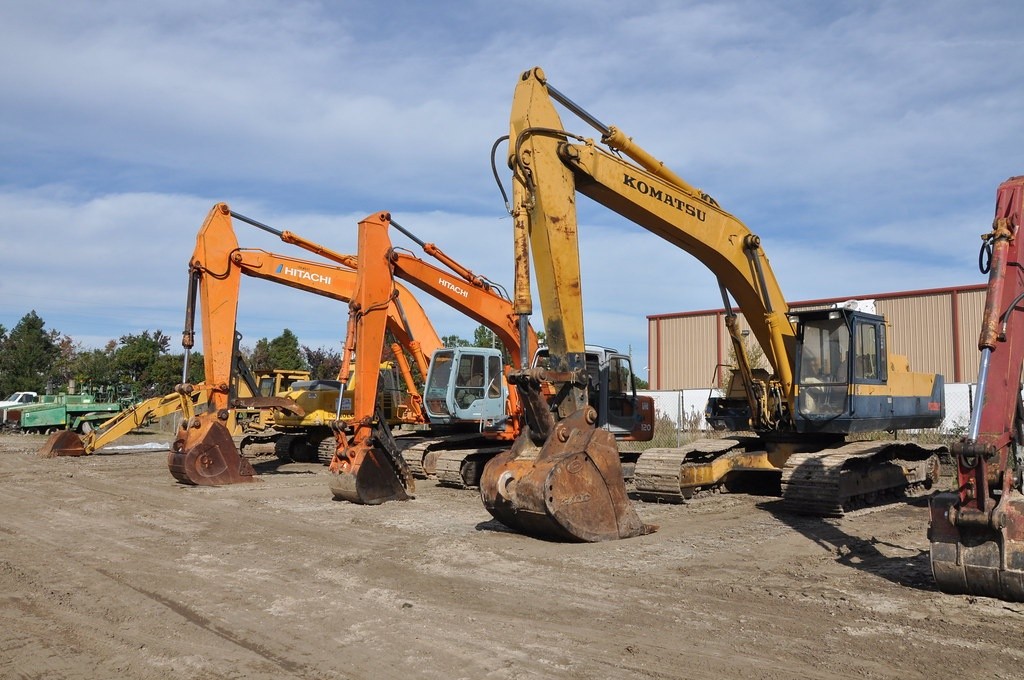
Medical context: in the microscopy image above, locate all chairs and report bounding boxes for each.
[829,358,867,405]
[455,374,484,407]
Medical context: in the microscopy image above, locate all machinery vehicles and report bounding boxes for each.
[928,175,1024,605]
[328,210,654,508]
[0,329,310,460]
[479,66,957,541]
[229,328,410,470]
[166,201,523,492]
